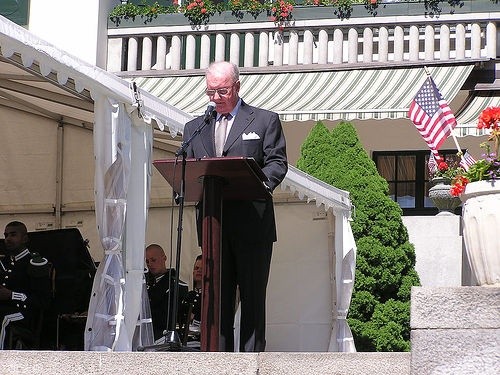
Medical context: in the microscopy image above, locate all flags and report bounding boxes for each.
[428,152,437,176]
[409,77,457,154]
[460,152,477,171]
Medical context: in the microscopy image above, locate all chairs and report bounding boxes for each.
[4,262,97,351]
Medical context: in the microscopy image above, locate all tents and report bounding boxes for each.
[0,14,357,352]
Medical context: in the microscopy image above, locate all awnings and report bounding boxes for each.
[118,63,478,124]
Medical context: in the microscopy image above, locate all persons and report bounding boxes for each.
[139,244,188,339]
[0,221,67,350]
[182,61,288,353]
[178,255,203,334]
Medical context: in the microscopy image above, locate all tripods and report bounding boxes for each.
[137,121,211,355]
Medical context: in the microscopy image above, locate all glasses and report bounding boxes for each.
[205,79,239,97]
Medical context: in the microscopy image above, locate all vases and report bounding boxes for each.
[429,177,463,216]
[459,178,500,287]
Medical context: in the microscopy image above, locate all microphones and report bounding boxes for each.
[204,102,217,121]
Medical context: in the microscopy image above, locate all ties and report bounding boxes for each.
[214,113,230,157]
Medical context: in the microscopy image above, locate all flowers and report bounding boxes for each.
[173,0,378,32]
[435,105,500,197]
